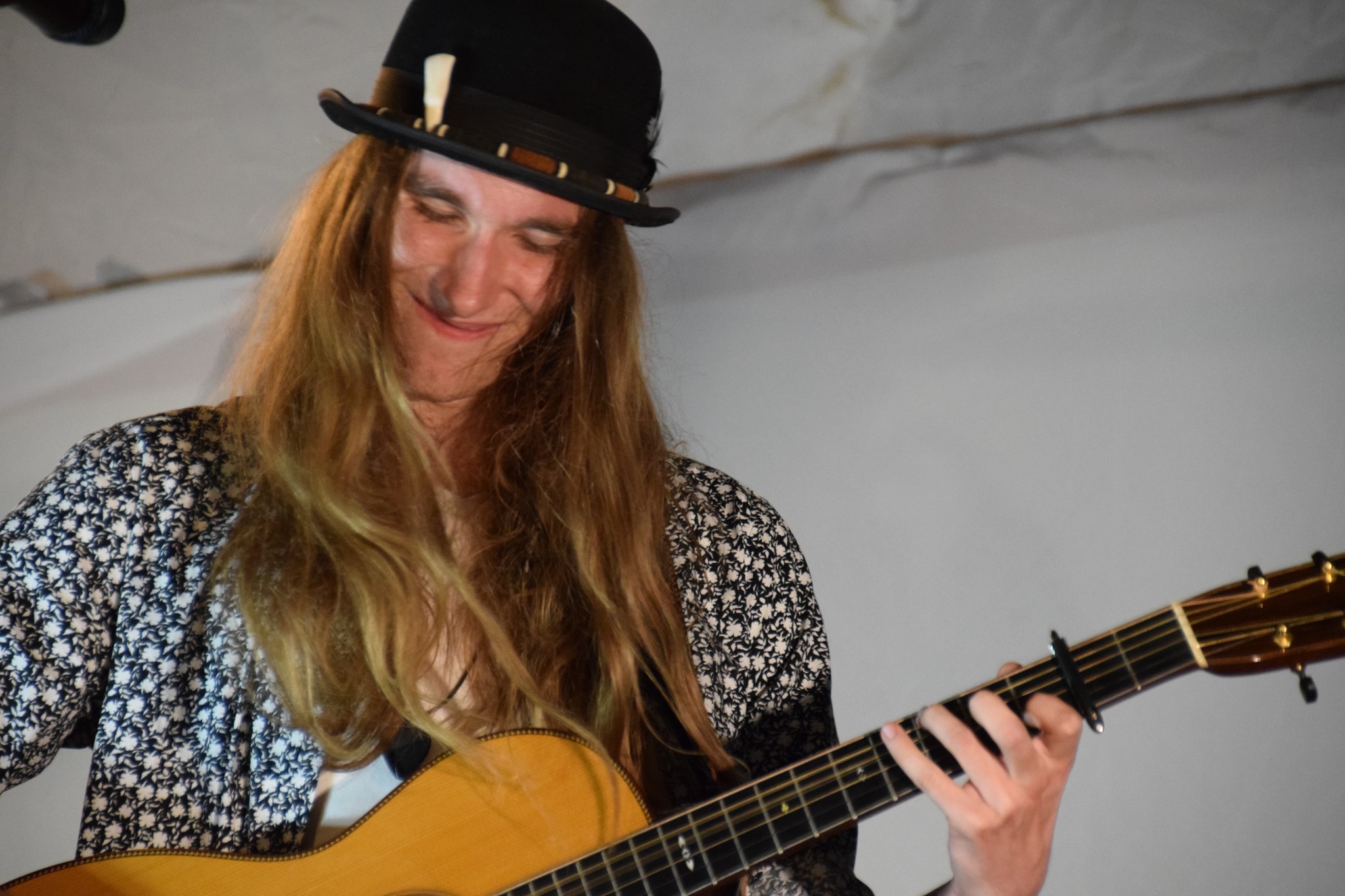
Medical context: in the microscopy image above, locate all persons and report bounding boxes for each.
[1,2,1085,896]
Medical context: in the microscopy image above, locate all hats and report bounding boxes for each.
[317,0,681,228]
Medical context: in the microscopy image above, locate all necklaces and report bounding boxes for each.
[379,637,486,780]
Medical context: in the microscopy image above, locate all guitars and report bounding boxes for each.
[0,549,1345,896]
